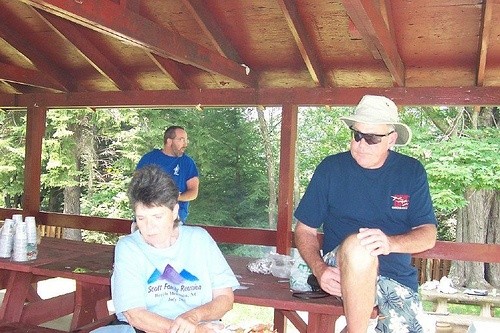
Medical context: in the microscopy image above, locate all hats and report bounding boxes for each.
[339,94,411,145]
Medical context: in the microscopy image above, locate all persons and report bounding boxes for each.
[111,166,240,333]
[130,126,198,234]
[294,95,437,333]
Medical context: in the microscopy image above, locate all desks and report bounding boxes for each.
[0,236,379,333]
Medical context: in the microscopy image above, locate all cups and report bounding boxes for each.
[0,215,37,261]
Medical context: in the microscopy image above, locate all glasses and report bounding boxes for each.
[349,125,397,145]
[174,139,190,144]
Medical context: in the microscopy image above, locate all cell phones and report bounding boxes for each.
[292,291,329,298]
